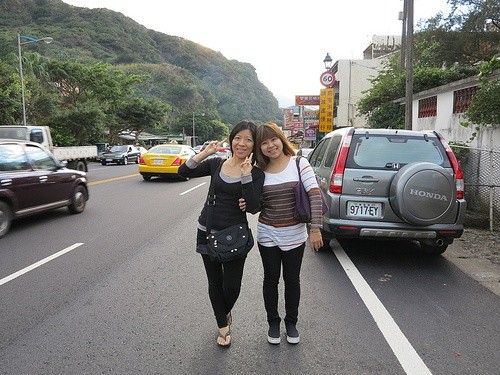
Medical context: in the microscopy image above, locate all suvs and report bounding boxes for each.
[0,139,90,239]
[301,125,467,255]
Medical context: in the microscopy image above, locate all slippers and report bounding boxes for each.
[216,327,231,348]
[227,312,232,325]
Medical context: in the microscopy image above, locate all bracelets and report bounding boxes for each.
[310,230,319,233]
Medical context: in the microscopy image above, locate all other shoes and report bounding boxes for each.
[286,324,300,344]
[267,321,280,344]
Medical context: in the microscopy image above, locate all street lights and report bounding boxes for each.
[192,111,205,147]
[16,34,53,126]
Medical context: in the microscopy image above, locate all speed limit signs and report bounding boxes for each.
[319,70,335,88]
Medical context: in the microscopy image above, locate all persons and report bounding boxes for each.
[177,120,266,348]
[239,122,323,344]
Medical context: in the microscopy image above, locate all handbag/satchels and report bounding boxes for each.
[206,224,248,263]
[293,157,329,221]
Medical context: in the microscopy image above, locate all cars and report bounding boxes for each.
[99,145,141,165]
[138,139,197,181]
[193,141,234,158]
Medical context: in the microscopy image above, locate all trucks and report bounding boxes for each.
[1,125,98,174]
